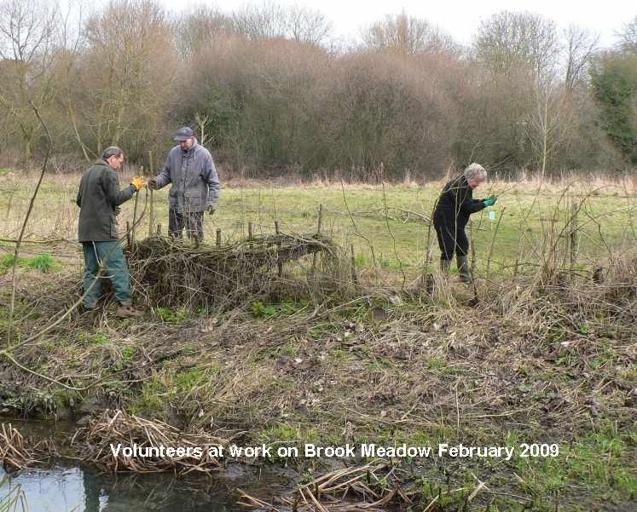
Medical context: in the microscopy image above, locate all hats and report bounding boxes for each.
[174,127,193,142]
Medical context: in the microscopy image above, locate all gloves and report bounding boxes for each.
[129,177,146,192]
[482,195,497,206]
[207,204,216,215]
[148,178,157,192]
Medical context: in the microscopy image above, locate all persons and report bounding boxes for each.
[432,162,497,307]
[76,146,145,313]
[147,126,221,247]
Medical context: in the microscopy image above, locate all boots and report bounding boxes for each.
[439,258,452,281]
[456,255,487,287]
[116,299,145,320]
